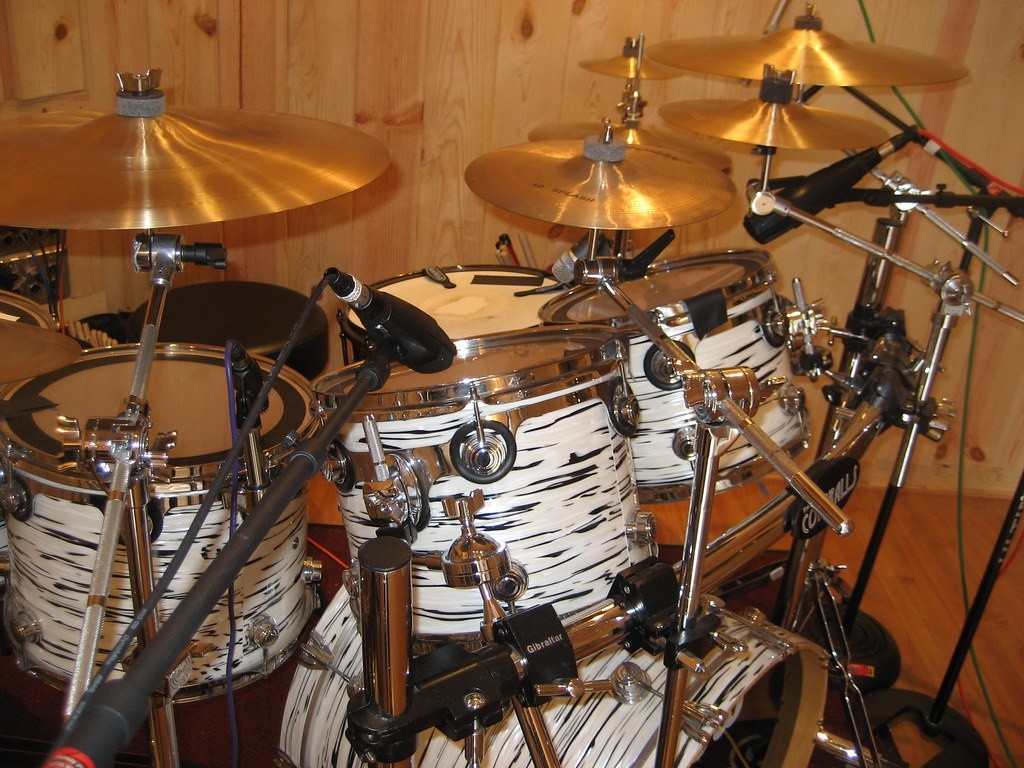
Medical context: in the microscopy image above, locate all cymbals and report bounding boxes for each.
[464,138,737,230]
[0,318,85,385]
[645,27,971,86]
[658,99,890,151]
[0,107,391,231]
[578,55,684,80]
[527,122,732,171]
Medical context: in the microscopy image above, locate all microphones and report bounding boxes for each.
[325,267,458,374]
[743,131,915,243]
[550,228,607,284]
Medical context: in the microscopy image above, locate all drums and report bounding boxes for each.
[273,582,831,768]
[0,289,57,603]
[0,342,322,704]
[307,324,659,657]
[340,264,574,364]
[537,246,811,504]
